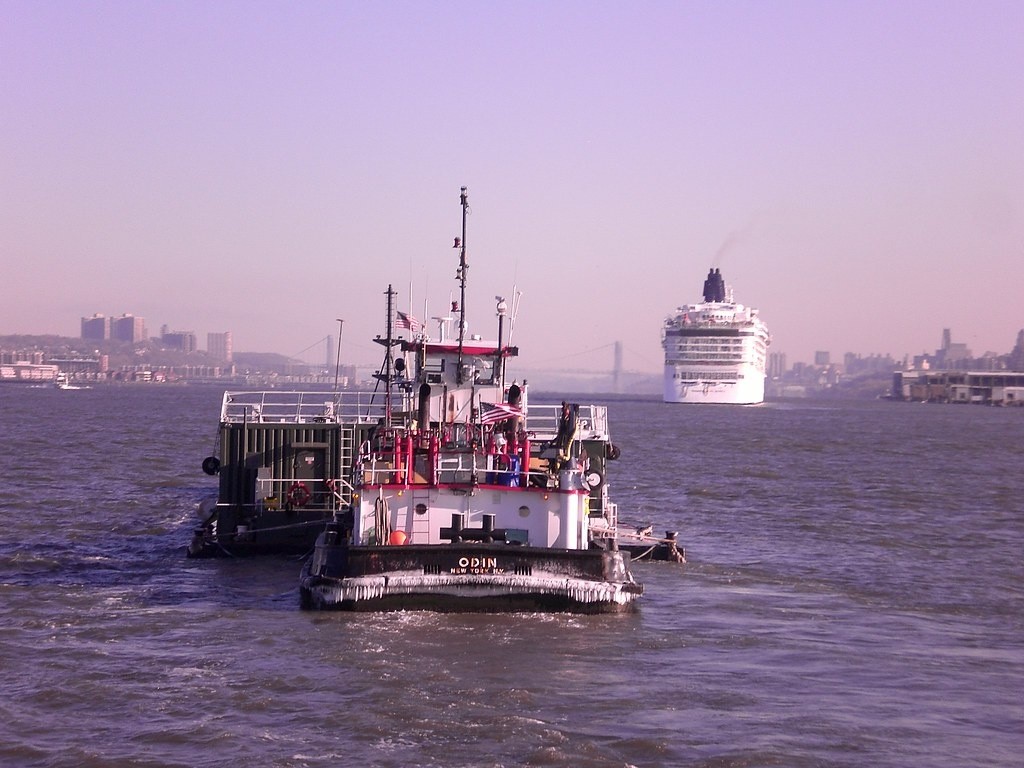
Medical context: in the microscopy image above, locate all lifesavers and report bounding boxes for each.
[287,484,311,506]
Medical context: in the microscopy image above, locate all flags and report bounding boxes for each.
[396,311,419,332]
[480,402,524,424]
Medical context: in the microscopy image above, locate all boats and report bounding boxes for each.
[189,186,686,613]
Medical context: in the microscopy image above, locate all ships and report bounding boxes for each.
[662,268,772,404]
